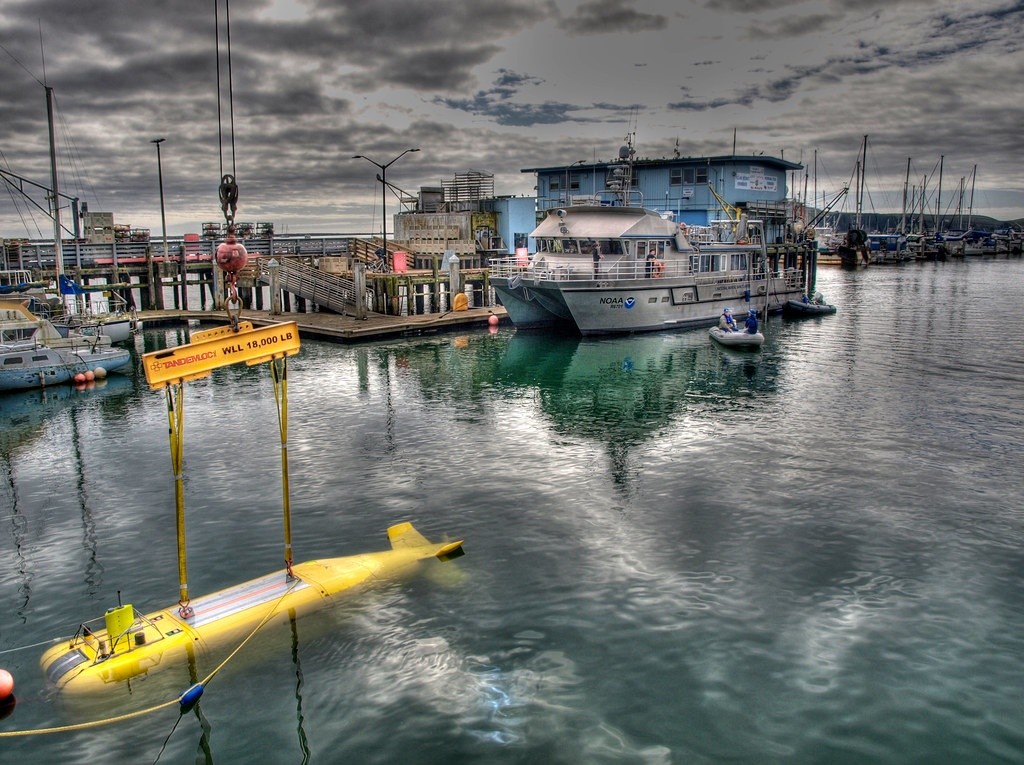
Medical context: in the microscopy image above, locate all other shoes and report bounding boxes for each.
[383,270,388,273]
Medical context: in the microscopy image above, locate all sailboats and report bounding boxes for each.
[781,134,1024,267]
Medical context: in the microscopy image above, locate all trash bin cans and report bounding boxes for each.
[392,252,407,273]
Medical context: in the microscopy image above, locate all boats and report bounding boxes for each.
[0,270,138,393]
[709,326,765,348]
[488,105,804,336]
[782,300,836,317]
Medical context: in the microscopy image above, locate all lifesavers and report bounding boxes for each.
[679,221,688,234]
[652,262,662,278]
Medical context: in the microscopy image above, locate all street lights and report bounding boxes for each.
[149,138,169,261]
[351,147,422,273]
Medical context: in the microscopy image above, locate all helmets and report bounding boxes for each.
[748,308,756,316]
[724,309,730,312]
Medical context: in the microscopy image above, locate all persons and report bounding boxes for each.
[719,308,739,334]
[745,309,758,334]
[588,243,605,279]
[645,249,656,278]
